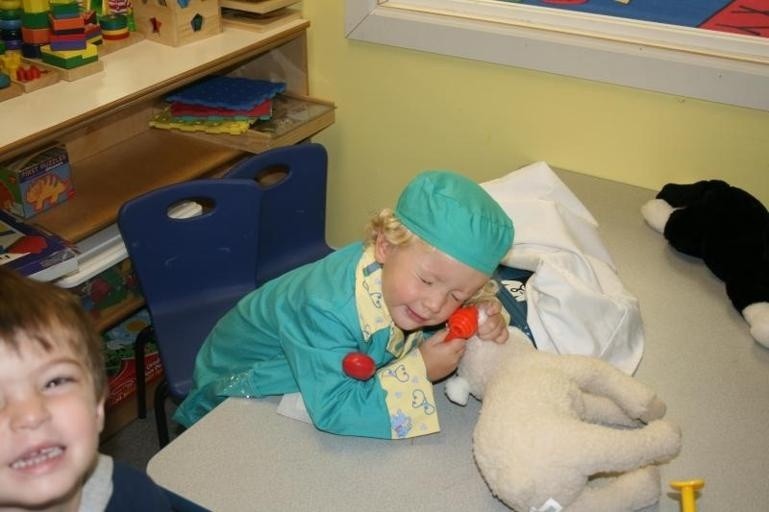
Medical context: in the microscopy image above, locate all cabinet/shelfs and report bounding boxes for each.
[1,17,313,443]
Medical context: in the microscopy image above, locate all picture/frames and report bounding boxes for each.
[339,0,769,113]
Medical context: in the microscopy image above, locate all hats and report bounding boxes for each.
[395,168,516,280]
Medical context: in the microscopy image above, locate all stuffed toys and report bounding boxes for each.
[444,327,681,511]
[641,179,769,349]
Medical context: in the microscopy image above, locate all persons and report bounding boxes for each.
[170,170,516,439]
[1,266,215,512]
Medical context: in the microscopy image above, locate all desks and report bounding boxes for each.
[143,159,768,512]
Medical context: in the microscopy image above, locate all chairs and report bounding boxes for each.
[115,177,263,450]
[223,142,338,286]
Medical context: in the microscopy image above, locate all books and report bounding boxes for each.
[0,211,79,283]
[170,96,337,156]
